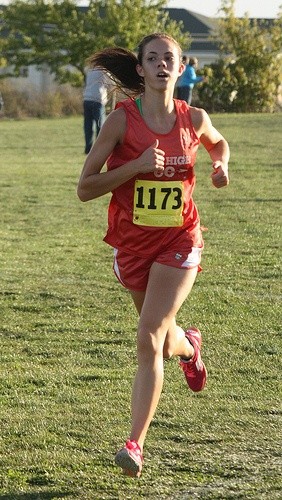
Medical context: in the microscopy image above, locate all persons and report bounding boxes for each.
[83,54,206,155]
[78,33,230,477]
